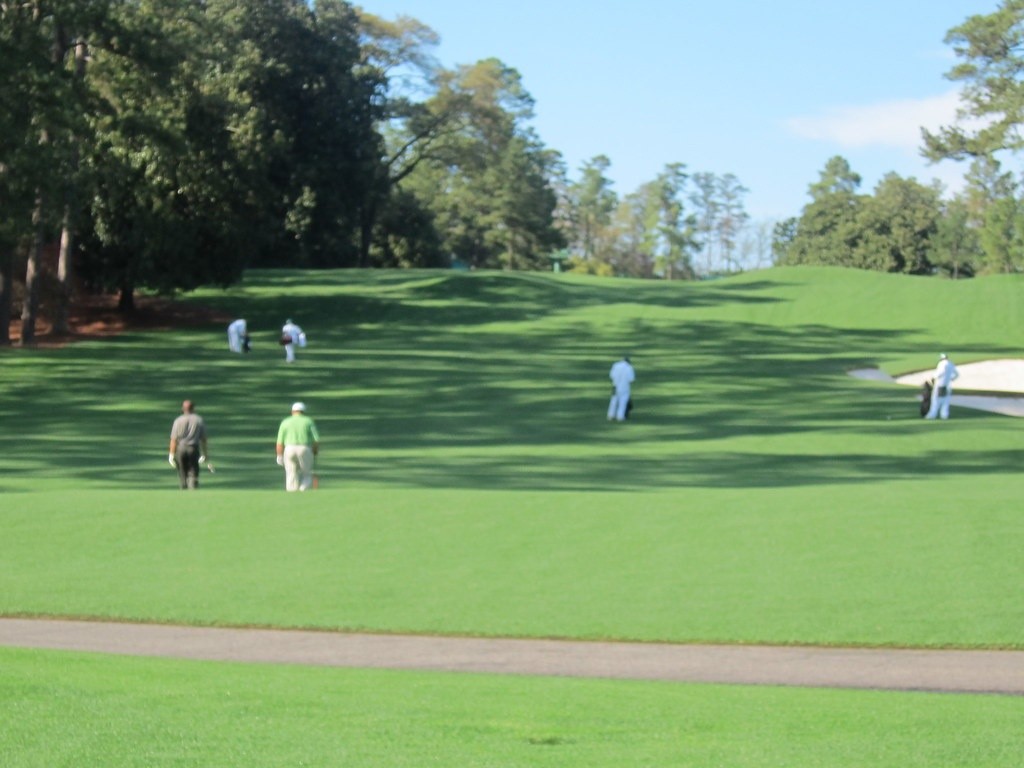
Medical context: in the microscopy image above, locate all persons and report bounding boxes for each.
[275,400,320,492]
[923,353,959,419]
[169,400,208,490]
[606,354,636,422]
[283,320,307,362]
[227,318,247,353]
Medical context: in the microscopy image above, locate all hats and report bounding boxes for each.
[939,354,946,360]
[292,401,305,411]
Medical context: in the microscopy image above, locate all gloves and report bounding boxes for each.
[199,455,205,465]
[275,455,283,466]
[168,454,175,467]
